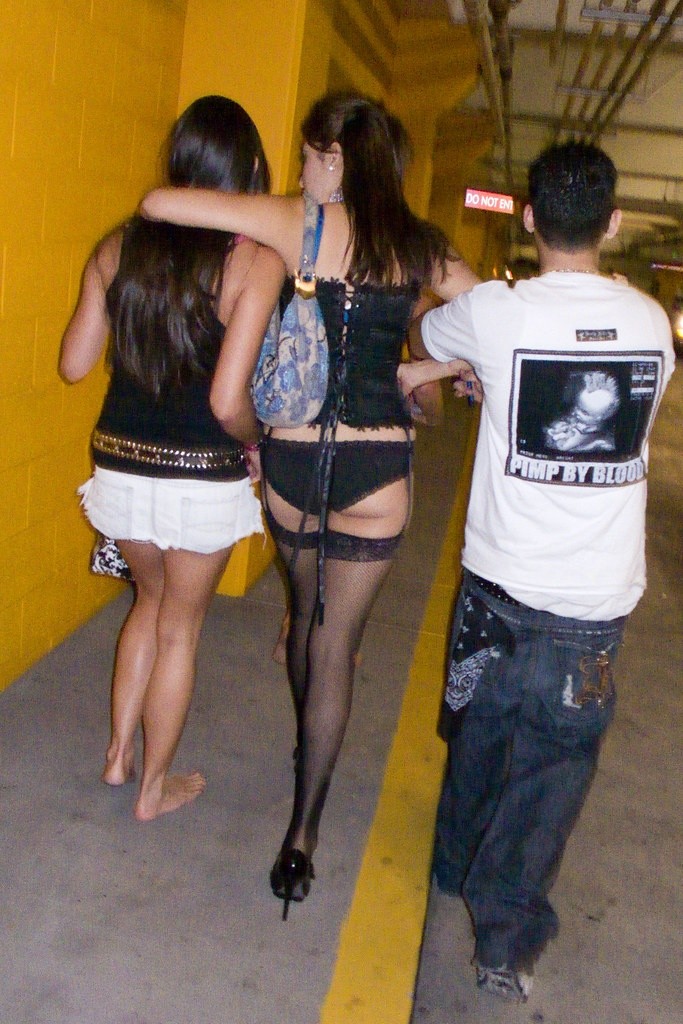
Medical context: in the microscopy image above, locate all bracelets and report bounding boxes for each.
[242,443,261,452]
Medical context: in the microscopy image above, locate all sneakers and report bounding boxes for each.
[473,959,537,1006]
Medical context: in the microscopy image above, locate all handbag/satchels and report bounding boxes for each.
[246,193,331,430]
[87,528,136,582]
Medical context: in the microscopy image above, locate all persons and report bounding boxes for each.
[405,140,676,1003]
[132,91,489,918]
[53,93,286,826]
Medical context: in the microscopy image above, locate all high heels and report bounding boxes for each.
[267,845,317,923]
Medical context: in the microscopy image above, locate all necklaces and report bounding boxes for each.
[540,269,596,275]
